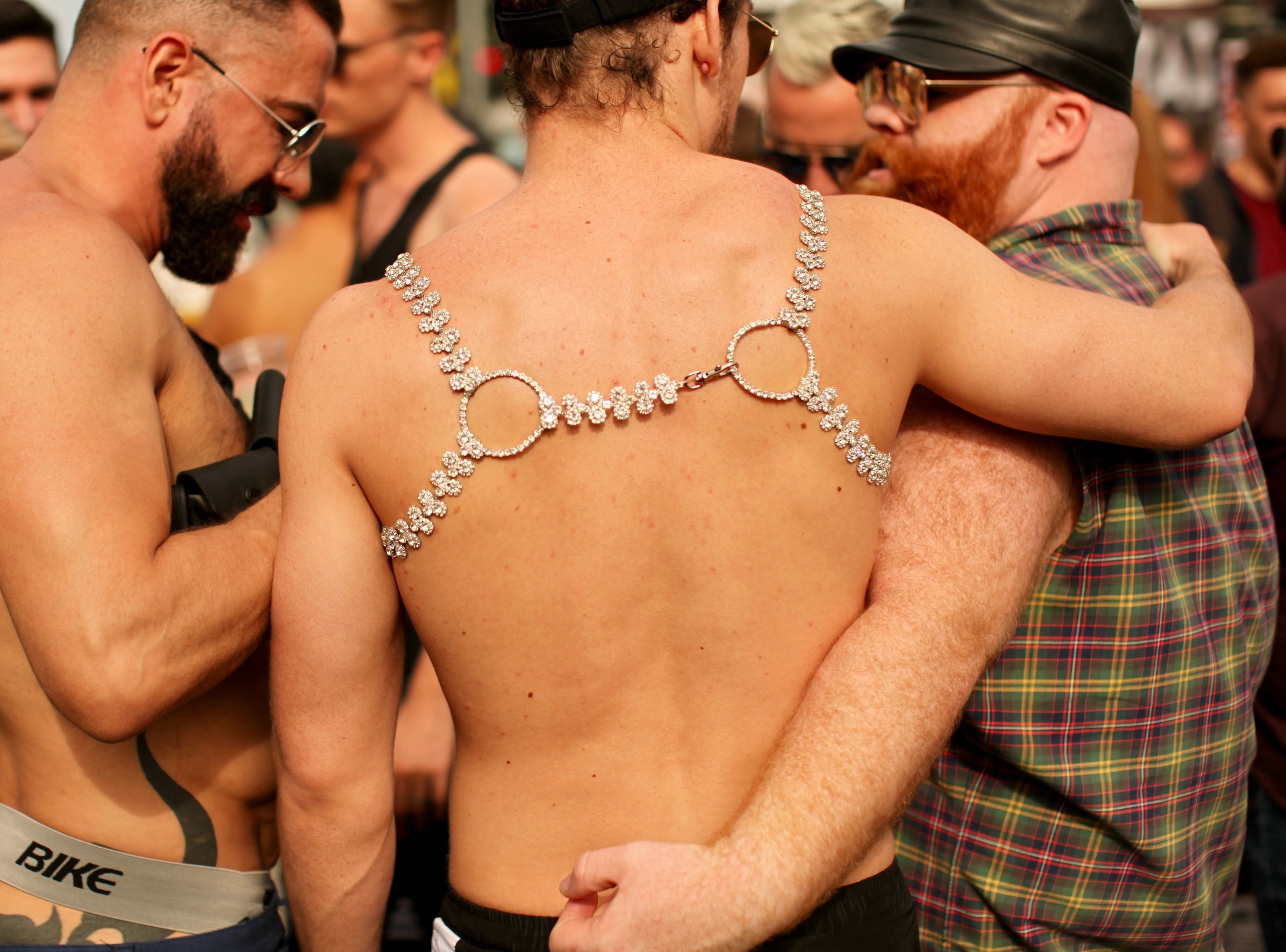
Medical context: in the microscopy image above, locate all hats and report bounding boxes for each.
[834,1,1141,117]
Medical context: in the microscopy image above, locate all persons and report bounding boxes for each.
[171,0,521,422]
[0,1,60,163]
[273,0,1286,952]
[0,0,344,952]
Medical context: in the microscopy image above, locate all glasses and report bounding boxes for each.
[331,24,428,73]
[767,146,853,188]
[142,47,325,172]
[854,62,1065,126]
[724,1,778,75]
